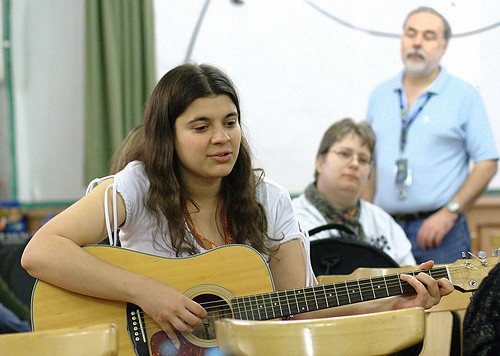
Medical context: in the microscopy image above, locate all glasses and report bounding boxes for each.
[328,144,373,169]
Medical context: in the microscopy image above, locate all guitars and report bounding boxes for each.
[31,239,500,356]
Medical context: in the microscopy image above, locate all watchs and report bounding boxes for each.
[447,202,461,216]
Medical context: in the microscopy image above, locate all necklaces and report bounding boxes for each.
[183,204,232,249]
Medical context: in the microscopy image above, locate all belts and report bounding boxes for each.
[390,209,443,221]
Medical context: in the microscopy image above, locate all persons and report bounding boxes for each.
[289,118,416,269]
[365,6,500,267]
[21,64,454,356]
